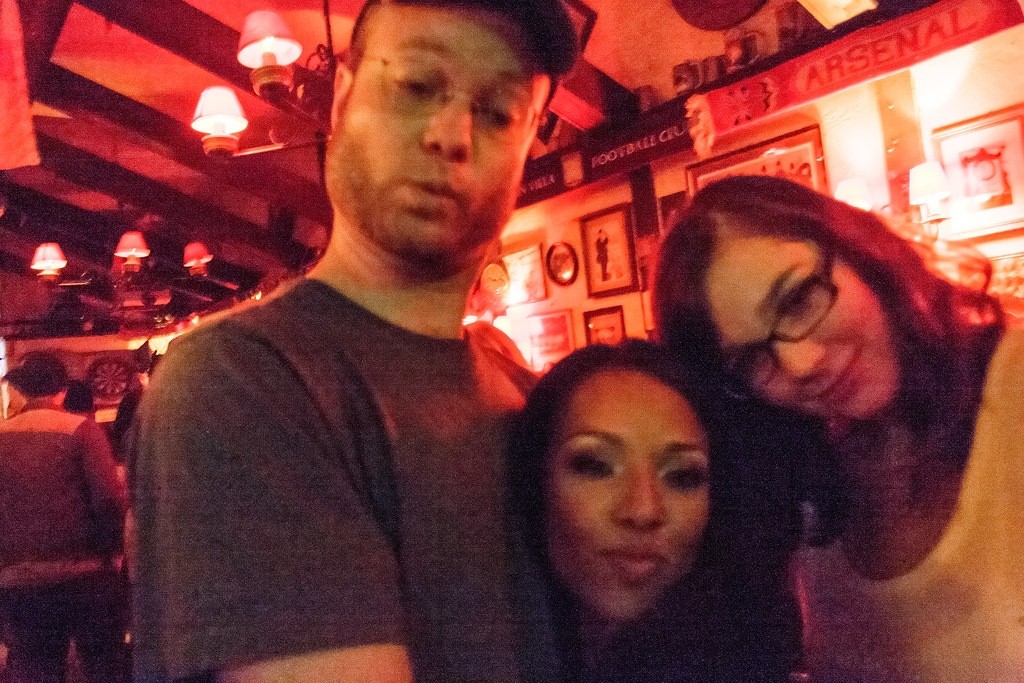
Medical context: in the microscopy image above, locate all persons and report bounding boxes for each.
[507,339,805,683]
[123,0,587,683]
[654,175,1024,683]
[0,352,147,683]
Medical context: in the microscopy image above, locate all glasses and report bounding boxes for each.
[323,53,552,145]
[708,239,842,403]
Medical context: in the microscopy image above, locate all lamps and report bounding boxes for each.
[30,187,215,321]
[191,0,337,165]
[833,159,954,244]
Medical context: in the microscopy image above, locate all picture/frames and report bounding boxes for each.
[684,121,830,204]
[576,199,638,299]
[929,102,1024,247]
[581,304,627,346]
[544,242,580,287]
[523,243,547,306]
[527,307,576,364]
